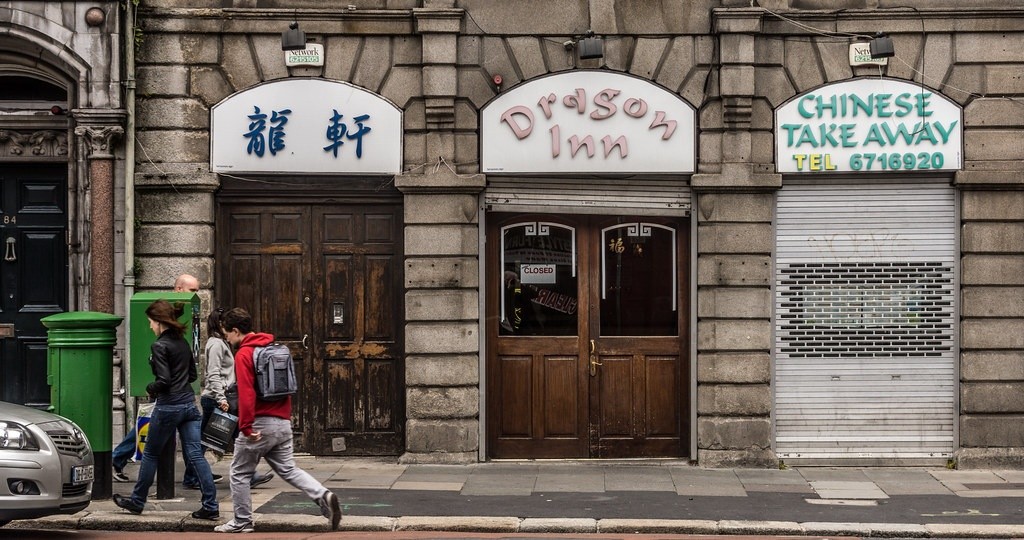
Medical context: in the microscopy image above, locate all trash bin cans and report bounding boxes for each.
[40,311,124,499]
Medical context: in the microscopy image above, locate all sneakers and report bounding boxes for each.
[192,507,220,519]
[212,473,223,482]
[250,474,274,488]
[112,465,129,482]
[183,480,202,489]
[113,494,142,515]
[214,518,254,533]
[321,491,342,530]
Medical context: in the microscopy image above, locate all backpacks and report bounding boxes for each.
[253,341,298,402]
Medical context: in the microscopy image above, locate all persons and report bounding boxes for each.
[112,274,223,484]
[214,307,341,532]
[505,271,527,334]
[112,301,219,520]
[199,310,274,488]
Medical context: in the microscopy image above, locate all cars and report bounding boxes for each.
[0,400,95,525]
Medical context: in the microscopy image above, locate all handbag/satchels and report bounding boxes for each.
[131,396,157,463]
[199,407,239,453]
